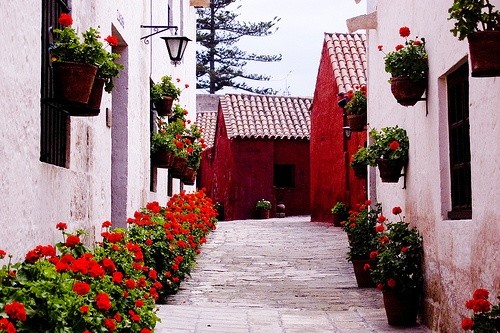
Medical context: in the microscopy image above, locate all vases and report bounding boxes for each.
[390,75,427,106]
[259,210,269,219]
[376,158,404,182]
[381,289,420,328]
[467,30,500,76]
[156,149,197,185]
[353,162,367,177]
[69,78,105,109]
[52,61,97,104]
[348,114,365,131]
[153,96,172,116]
[351,256,379,288]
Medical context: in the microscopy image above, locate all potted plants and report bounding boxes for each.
[331,201,346,228]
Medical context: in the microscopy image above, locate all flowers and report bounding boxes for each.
[49,13,124,94]
[340,0,500,333]
[256,199,271,209]
[0,187,219,333]
[152,75,207,169]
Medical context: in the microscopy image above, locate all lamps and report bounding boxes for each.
[140,25,193,66]
